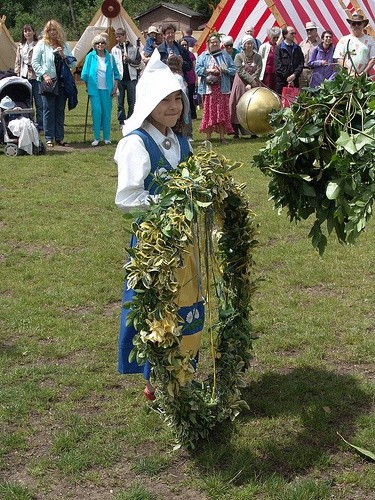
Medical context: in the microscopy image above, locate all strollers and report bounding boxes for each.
[0,70,46,158]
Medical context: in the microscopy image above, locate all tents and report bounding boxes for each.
[192,0,375,58]
[69,0,146,73]
[0,14,19,72]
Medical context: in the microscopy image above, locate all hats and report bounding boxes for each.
[147,26,158,35]
[305,21,317,29]
[0,96,15,109]
[346,9,369,27]
[242,35,255,49]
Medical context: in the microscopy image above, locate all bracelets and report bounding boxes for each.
[43,74,48,78]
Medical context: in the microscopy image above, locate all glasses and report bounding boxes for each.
[225,44,233,47]
[351,21,362,27]
[96,43,104,44]
[326,35,332,38]
[291,32,297,35]
[273,37,279,39]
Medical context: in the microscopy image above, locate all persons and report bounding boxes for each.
[0,7,375,147]
[118,43,206,401]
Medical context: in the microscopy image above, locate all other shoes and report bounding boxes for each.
[202,139,212,144]
[220,139,228,144]
[233,136,239,140]
[104,140,111,144]
[46,141,54,147]
[143,390,156,400]
[92,141,98,146]
[187,135,194,141]
[59,142,68,146]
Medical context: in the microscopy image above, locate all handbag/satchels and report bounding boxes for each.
[28,65,33,71]
[281,81,299,107]
[37,79,59,95]
[193,87,201,105]
